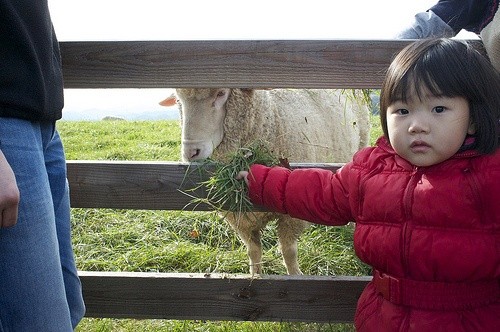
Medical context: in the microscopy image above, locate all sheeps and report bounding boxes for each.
[158,88,371,274]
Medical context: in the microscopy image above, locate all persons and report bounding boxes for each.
[0,0,86,332]
[236,38,500,331]
[396,0,500,76]
